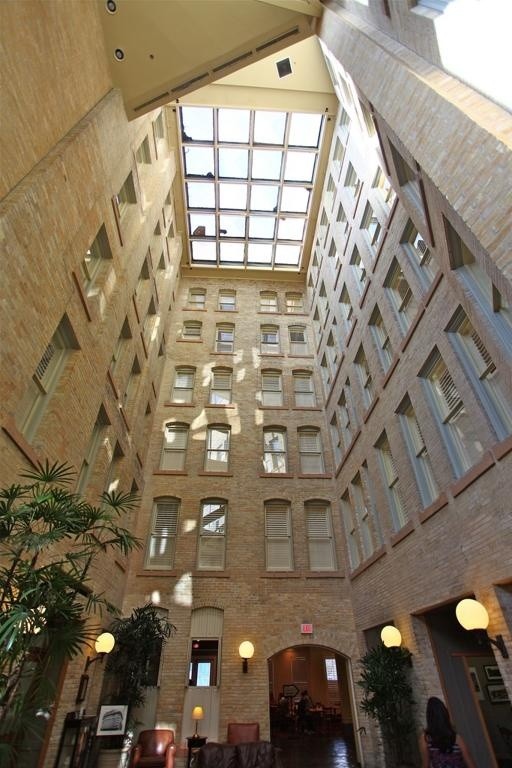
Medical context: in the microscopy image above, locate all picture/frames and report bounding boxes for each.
[95,703,129,737]
[469,664,510,705]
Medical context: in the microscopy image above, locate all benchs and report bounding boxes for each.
[190,742,283,768]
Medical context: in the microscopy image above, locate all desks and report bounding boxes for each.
[53,711,98,768]
[185,735,207,768]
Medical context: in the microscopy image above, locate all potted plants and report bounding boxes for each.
[96,603,176,767]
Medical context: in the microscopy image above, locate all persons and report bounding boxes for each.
[420,696,476,766]
[269,688,323,736]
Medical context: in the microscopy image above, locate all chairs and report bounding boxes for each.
[130,729,177,768]
[226,721,259,746]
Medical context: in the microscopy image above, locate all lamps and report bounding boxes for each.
[84,631,116,674]
[456,597,508,660]
[238,640,254,673]
[191,705,203,738]
[380,625,412,657]
